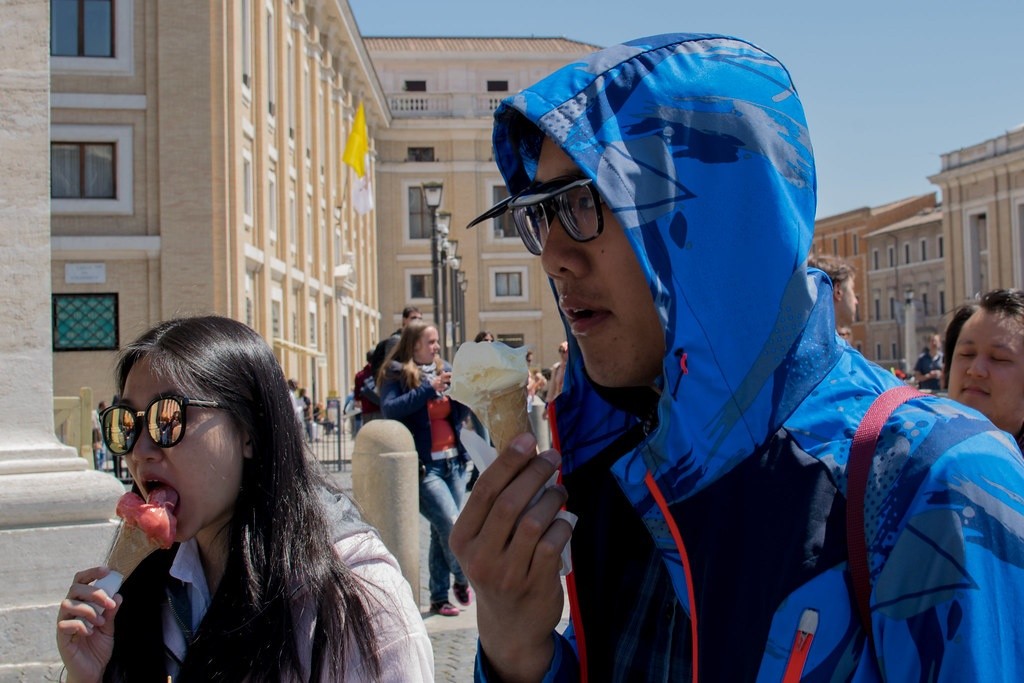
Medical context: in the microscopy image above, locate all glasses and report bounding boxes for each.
[466,179,605,256]
[558,349,565,353]
[484,338,494,343]
[411,316,422,320]
[99,394,230,456]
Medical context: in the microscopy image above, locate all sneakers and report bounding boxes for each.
[430,601,459,616]
[453,583,472,606]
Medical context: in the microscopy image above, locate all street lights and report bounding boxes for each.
[421,179,443,358]
[436,210,467,362]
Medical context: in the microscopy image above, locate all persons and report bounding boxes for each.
[55,316,468,683]
[450,32,1024,682]
[343,307,497,616]
[164,411,182,443]
[288,379,335,443]
[92,400,109,470]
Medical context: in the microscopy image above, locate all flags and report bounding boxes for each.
[342,100,369,178]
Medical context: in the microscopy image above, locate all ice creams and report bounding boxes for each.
[102,489,177,584]
[443,341,538,473]
